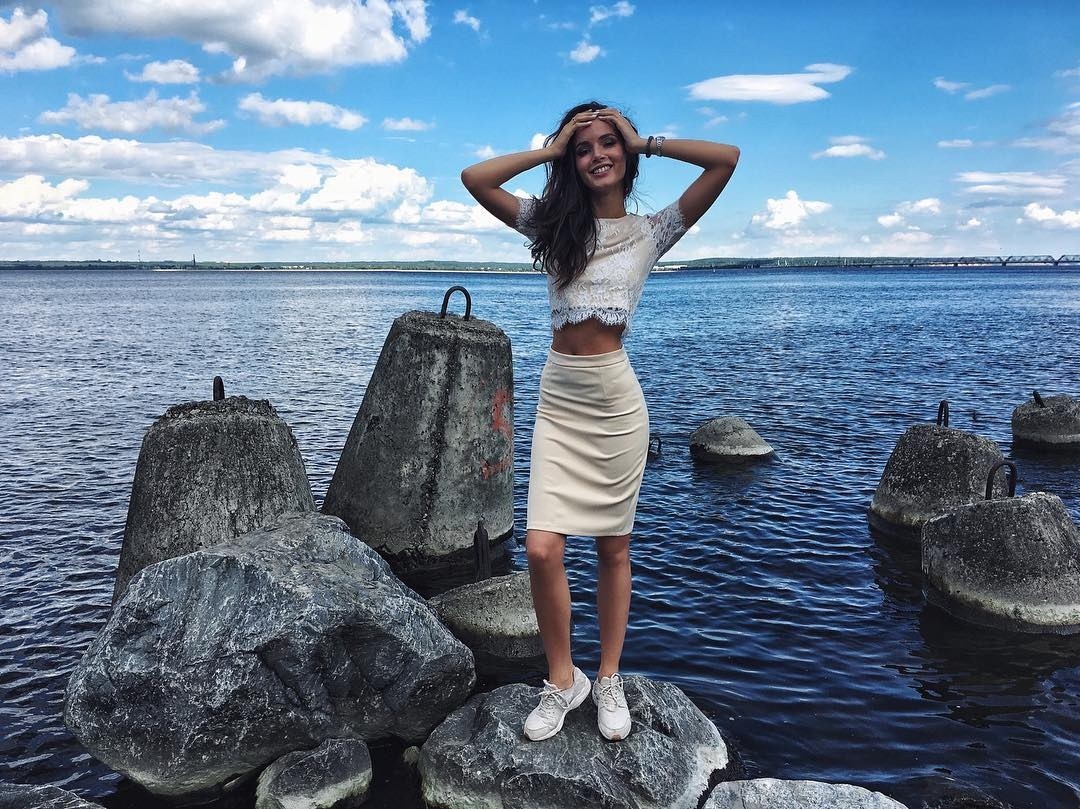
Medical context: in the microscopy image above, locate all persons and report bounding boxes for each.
[462,102,741,742]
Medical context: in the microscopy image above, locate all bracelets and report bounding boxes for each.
[646,136,654,159]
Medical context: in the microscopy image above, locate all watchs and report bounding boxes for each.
[655,136,665,157]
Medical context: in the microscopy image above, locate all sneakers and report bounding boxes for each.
[592,673,631,741]
[524,665,591,742]
